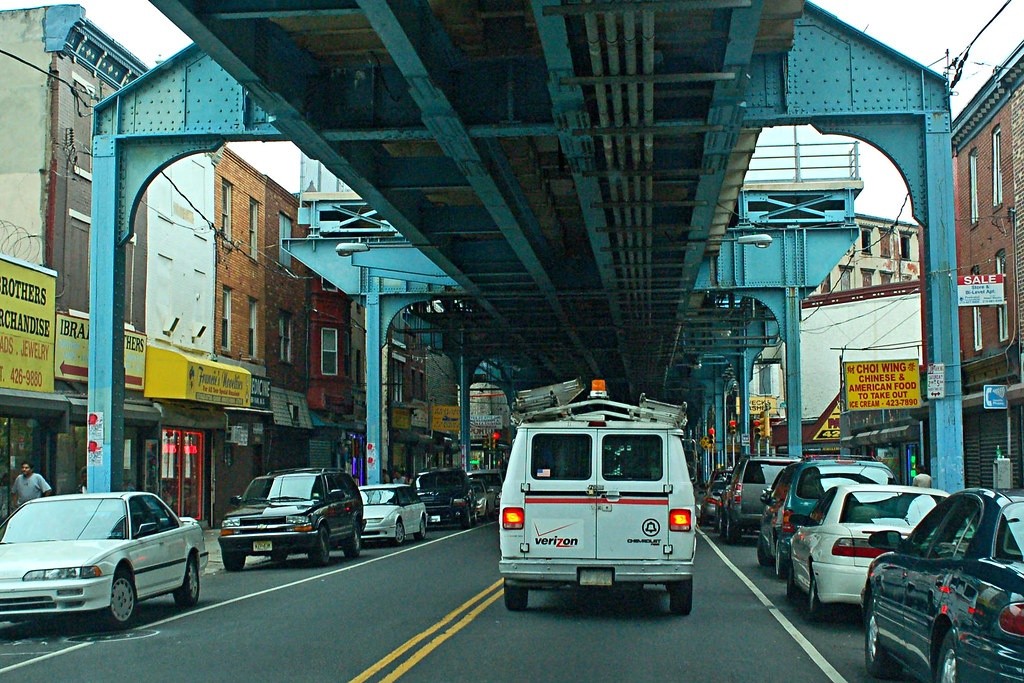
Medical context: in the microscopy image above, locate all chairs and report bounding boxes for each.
[845,506,880,523]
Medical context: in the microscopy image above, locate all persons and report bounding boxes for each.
[11,461,52,509]
[383,468,408,485]
[911,464,931,488]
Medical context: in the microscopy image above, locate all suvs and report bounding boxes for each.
[755,450,901,581]
[712,454,813,543]
[403,467,483,530]
[216,467,366,572]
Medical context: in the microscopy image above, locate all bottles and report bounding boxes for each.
[996,445,1001,460]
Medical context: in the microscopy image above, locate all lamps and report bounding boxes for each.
[708,234,773,248]
[335,243,413,256]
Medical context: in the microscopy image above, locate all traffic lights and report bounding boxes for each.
[708,428,715,445]
[752,420,763,440]
[759,410,771,439]
[729,420,736,435]
[489,431,500,449]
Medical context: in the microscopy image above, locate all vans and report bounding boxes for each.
[494,377,701,615]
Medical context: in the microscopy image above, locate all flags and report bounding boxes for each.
[536,469,550,476]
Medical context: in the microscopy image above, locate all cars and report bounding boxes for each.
[358,482,428,547]
[696,467,735,524]
[863,486,1024,683]
[785,480,977,621]
[468,468,506,523]
[0,491,210,630]
